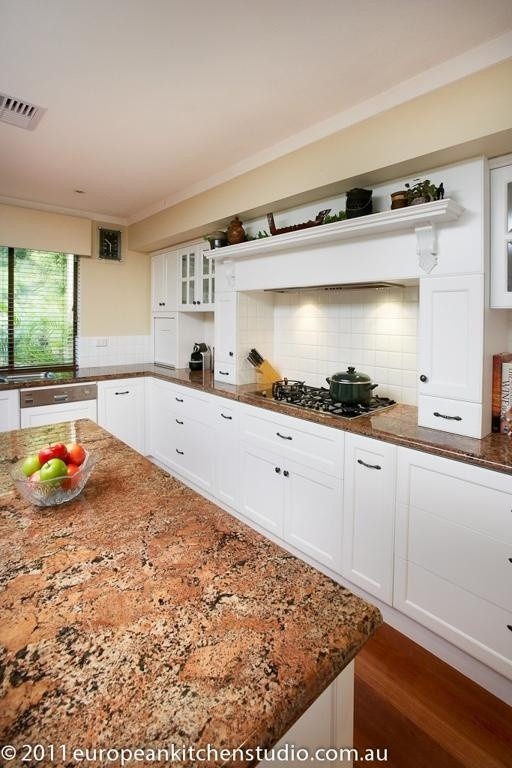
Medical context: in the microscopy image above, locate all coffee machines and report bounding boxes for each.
[189,340,213,373]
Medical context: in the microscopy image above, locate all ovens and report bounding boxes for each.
[18,384,99,428]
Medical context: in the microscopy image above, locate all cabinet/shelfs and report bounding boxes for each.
[213,393,237,520]
[150,315,206,368]
[98,377,147,458]
[150,250,180,312]
[148,376,216,502]
[415,276,508,438]
[0,390,21,431]
[398,443,512,684]
[487,164,512,311]
[234,404,344,584]
[213,291,275,388]
[345,435,397,615]
[177,243,215,313]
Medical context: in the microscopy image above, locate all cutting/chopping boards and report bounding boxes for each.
[262,281,405,294]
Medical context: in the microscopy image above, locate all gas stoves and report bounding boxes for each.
[245,377,400,422]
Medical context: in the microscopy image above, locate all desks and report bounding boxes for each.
[1,412,385,767]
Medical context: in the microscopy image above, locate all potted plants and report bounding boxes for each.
[402,178,445,206]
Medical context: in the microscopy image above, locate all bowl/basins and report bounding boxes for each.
[8,448,100,510]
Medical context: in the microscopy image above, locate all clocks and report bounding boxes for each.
[96,224,125,263]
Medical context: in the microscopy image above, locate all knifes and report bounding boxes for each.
[246,348,264,370]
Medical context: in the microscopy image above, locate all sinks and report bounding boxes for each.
[0,370,56,385]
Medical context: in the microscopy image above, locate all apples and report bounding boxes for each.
[21,441,86,491]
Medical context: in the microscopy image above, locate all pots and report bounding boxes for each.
[325,366,378,405]
